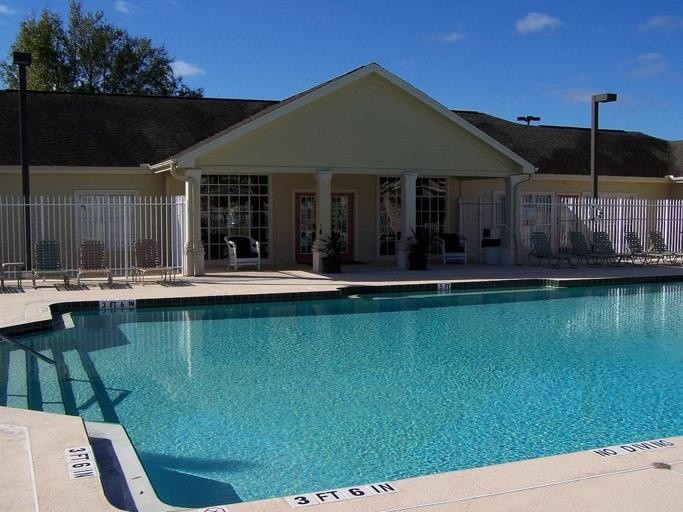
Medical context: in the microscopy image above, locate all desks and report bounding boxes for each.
[480,239,501,264]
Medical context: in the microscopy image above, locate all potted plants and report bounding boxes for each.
[410,227,428,270]
[318,230,342,273]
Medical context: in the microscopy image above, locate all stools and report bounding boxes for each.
[0,261,25,289]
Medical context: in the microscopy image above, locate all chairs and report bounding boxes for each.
[32,240,69,289]
[77,240,112,286]
[438,233,468,264]
[224,235,261,270]
[529,231,683,267]
[129,238,174,283]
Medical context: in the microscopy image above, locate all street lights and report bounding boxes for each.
[514,116,542,127]
[9,47,35,270]
[587,91,617,198]
